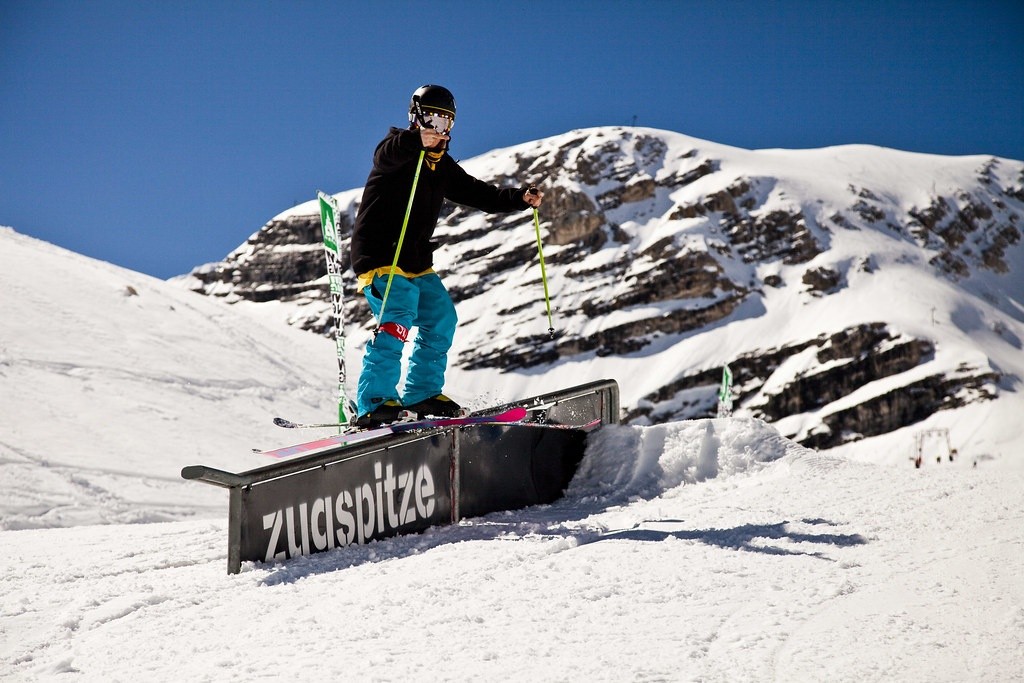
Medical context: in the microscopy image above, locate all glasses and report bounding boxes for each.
[411,113,454,132]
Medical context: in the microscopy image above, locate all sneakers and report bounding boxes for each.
[359,400,416,426]
[407,394,461,418]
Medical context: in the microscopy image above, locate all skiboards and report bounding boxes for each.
[250,406,603,457]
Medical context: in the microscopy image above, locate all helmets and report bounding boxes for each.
[410,84,456,119]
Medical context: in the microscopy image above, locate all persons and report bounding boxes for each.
[349,84,544,422]
